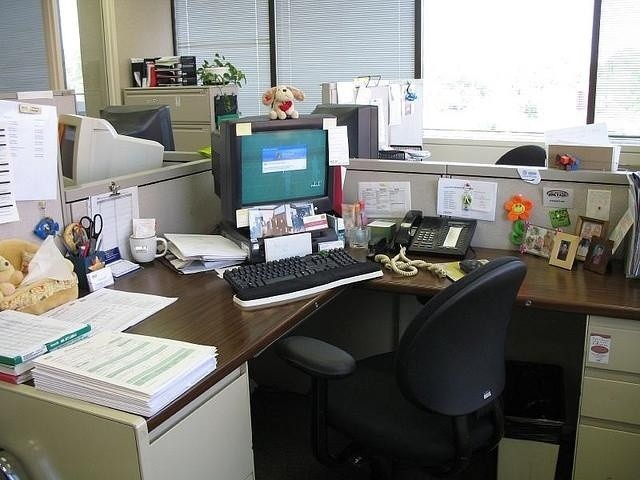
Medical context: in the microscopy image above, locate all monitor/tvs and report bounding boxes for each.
[58,114,165,187]
[210,114,337,227]
[100,103,175,152]
[311,104,379,161]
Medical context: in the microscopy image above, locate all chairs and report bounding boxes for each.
[278,255,528,480]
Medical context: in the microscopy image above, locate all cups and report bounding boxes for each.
[349,226,371,249]
[129,233,168,262]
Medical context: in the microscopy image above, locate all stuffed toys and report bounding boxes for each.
[262,85,305,120]
[2,255,23,295]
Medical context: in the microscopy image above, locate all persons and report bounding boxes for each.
[579,218,591,260]
[586,246,609,267]
[556,240,570,259]
[521,226,554,255]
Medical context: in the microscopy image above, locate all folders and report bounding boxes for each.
[626,172,638,279]
[130,56,197,87]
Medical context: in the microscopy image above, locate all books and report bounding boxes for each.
[0,306,90,387]
[46,329,219,417]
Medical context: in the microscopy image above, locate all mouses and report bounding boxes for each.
[459,259,483,273]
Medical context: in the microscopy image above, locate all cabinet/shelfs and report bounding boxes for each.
[121,84,239,153]
[564,306,640,476]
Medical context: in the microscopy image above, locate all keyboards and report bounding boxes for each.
[223,246,384,308]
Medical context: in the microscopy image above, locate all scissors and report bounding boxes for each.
[80,213,104,249]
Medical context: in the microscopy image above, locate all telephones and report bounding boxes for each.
[387,210,477,258]
